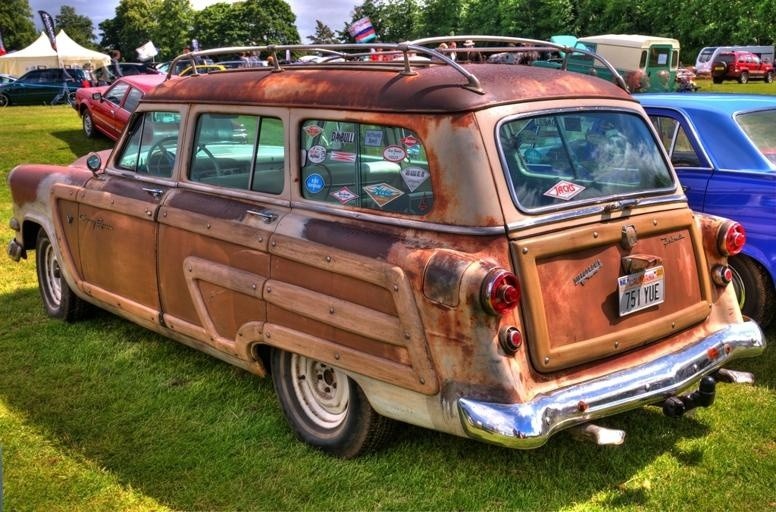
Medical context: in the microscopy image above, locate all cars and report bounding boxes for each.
[93,52,274,80]
[0,74,18,86]
[6,34,766,460]
[523,92,776,336]
[72,74,249,150]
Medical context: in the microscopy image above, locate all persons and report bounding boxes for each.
[239,50,274,69]
[431,39,540,68]
[80,50,123,88]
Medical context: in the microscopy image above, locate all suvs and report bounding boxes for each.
[0,68,108,106]
[711,51,775,84]
[529,35,681,94]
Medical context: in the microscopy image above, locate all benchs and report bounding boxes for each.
[209,160,436,217]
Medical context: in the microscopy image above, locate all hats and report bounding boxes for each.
[463,39,476,46]
